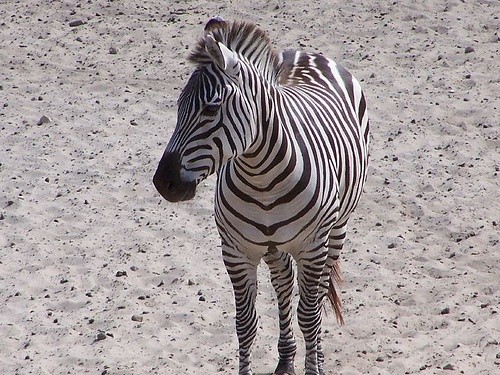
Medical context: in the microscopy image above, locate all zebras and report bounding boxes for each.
[153,19,372,374]
